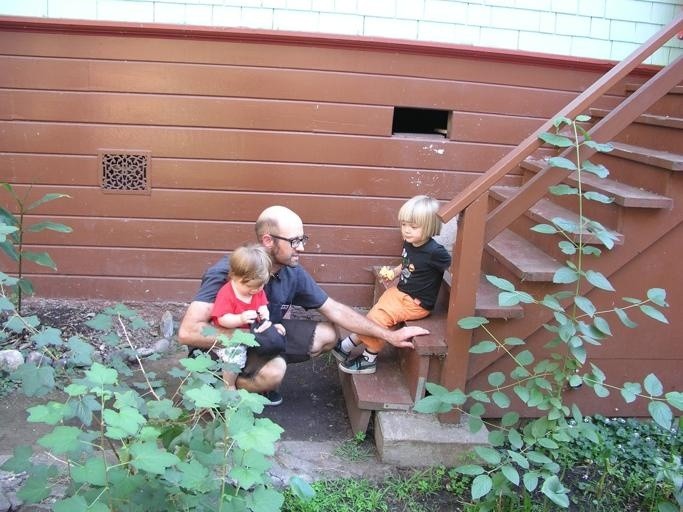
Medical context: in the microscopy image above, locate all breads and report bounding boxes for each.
[378,266,395,282]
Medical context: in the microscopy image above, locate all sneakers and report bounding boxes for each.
[259,390,283,406]
[331,338,351,362]
[339,354,377,375]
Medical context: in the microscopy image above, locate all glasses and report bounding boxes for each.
[270,234,309,248]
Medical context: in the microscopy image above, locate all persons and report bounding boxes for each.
[208,245,270,390]
[178,205,430,406]
[328,194,453,375]
